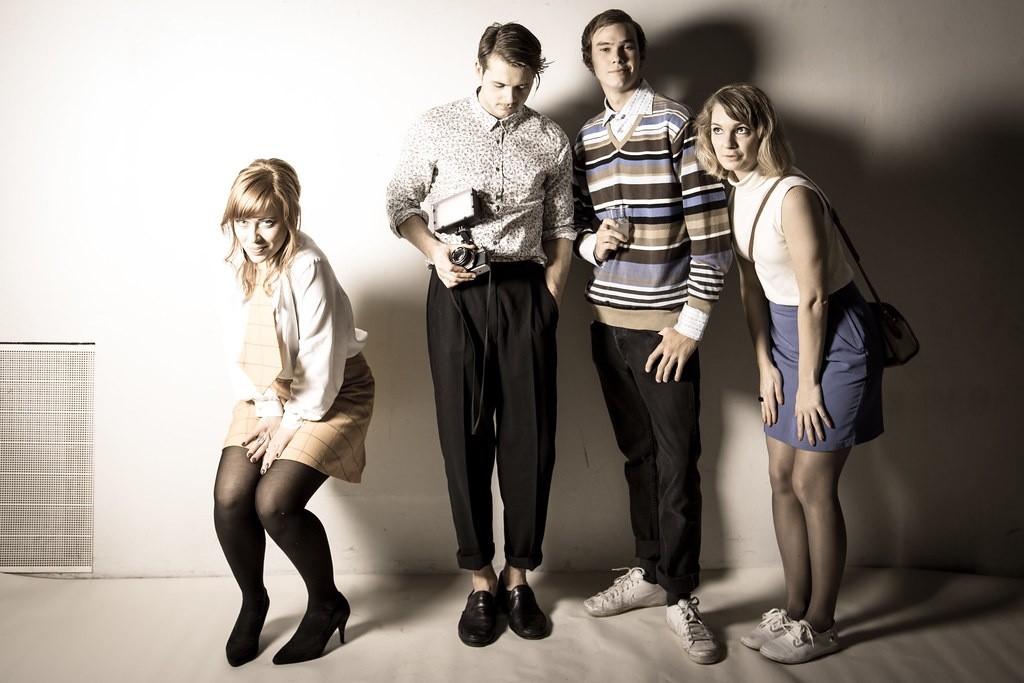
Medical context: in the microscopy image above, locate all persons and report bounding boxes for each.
[572,9,732,663]
[693,84,886,662]
[212,159,376,667]
[387,23,578,647]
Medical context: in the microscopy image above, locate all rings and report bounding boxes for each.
[258,433,266,443]
[758,397,764,403]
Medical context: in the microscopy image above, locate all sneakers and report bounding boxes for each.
[739,608,841,665]
[666,596,722,664]
[583,567,667,617]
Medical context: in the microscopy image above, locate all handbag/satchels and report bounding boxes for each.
[866,302,920,368]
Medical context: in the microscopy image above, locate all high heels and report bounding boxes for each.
[273,592,350,665]
[226,587,269,667]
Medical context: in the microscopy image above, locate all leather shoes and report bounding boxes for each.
[458,570,548,647]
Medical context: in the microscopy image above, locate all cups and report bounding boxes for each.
[605,205,629,242]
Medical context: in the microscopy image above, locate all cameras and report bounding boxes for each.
[450,246,491,276]
